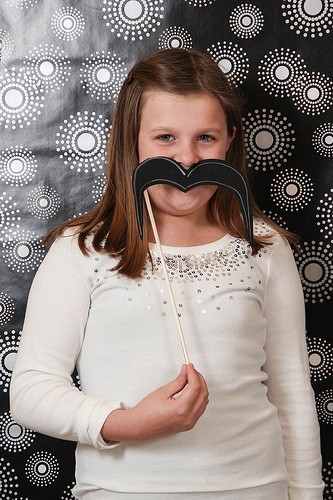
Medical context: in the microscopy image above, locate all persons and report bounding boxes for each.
[9,48,326,500]
